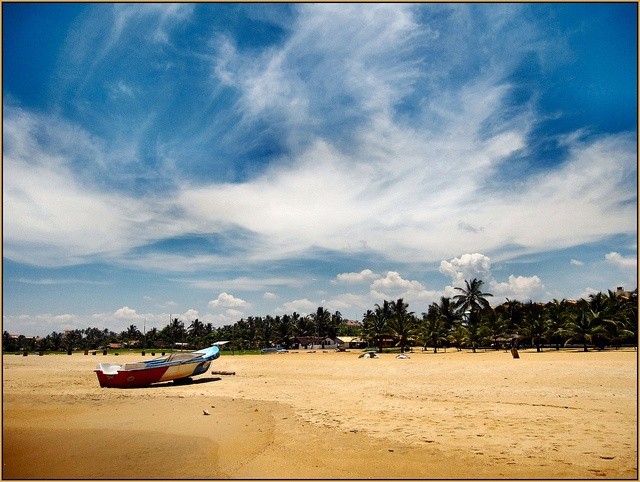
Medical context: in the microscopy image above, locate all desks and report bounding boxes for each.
[367,351,376,358]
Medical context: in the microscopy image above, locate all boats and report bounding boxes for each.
[93,345,221,389]
